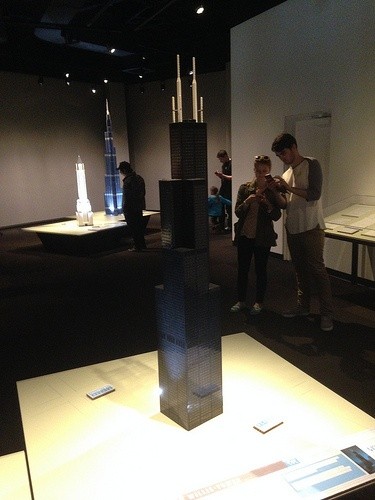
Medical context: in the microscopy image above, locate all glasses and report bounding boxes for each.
[255,156,269,159]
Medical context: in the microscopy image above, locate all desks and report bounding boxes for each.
[18,330,375,500]
[22,210,161,257]
[324,204,375,310]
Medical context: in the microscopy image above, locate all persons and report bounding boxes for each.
[264,133,333,331]
[207,150,231,231]
[115,161,147,253]
[230,155,281,315]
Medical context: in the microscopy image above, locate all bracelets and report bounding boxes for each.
[243,201,249,207]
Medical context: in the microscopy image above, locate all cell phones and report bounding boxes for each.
[264,174,274,182]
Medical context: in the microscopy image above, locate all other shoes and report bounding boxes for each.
[230,303,246,313]
[225,226,231,230]
[281,307,310,317]
[250,303,264,316]
[320,315,333,331]
[128,246,147,252]
